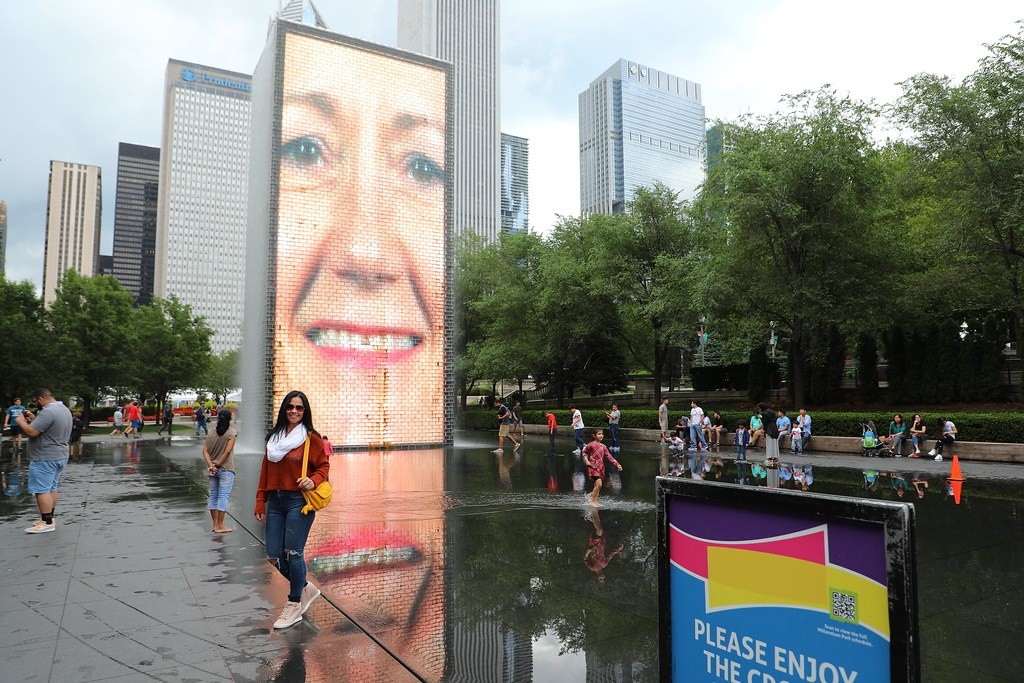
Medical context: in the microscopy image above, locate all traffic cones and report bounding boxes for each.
[946,481,966,505]
[946,455,966,480]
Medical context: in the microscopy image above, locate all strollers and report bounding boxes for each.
[859,421,893,458]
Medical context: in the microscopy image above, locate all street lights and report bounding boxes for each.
[698,316,705,367]
[770,320,779,362]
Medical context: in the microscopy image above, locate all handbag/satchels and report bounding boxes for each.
[301,480,333,515]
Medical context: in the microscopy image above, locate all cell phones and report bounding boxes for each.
[21,412,28,417]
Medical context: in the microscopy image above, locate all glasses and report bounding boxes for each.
[286,403,305,412]
[33,396,42,404]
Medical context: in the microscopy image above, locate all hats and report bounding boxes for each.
[117,406,123,410]
[200,402,205,405]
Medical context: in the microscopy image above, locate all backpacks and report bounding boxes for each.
[864,429,876,447]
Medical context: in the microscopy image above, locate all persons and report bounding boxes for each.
[157,401,174,435]
[883,414,957,459]
[583,428,623,498]
[583,508,624,583]
[274,446,450,683]
[110,399,143,439]
[204,396,223,414]
[69,412,83,459]
[15,387,73,533]
[890,470,956,500]
[659,455,724,480]
[688,400,708,450]
[203,410,238,533]
[734,420,749,462]
[674,411,728,448]
[748,407,812,454]
[254,390,329,629]
[272,28,450,442]
[196,402,208,436]
[322,436,334,461]
[758,403,780,462]
[735,461,813,492]
[4,398,27,452]
[661,432,685,457]
[490,399,621,453]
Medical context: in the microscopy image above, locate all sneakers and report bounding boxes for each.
[273,601,302,628]
[494,448,503,452]
[301,581,321,614]
[24,520,55,533]
[573,449,581,453]
[513,443,521,452]
[583,445,587,451]
[33,518,55,526]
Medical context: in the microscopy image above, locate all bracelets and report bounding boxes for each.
[213,463,220,469]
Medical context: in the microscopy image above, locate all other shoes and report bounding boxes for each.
[157,430,161,435]
[734,443,802,463]
[609,446,620,450]
[69,455,74,460]
[110,432,142,439]
[18,448,23,452]
[661,441,719,457]
[889,448,943,460]
[169,433,174,435]
[78,455,82,459]
